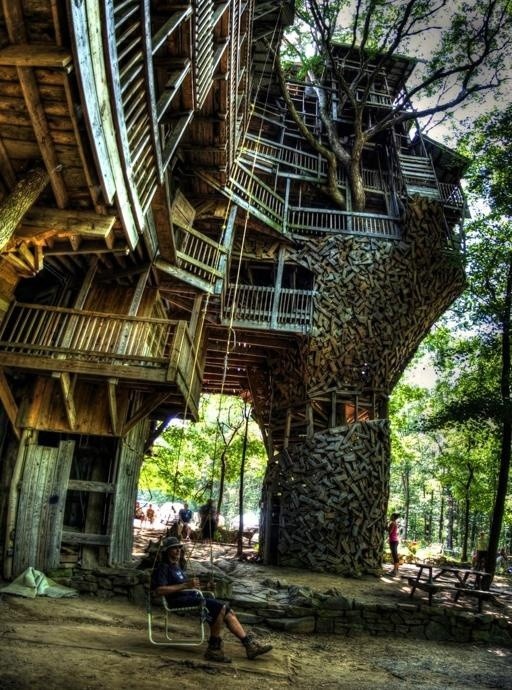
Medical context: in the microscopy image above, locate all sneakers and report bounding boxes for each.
[246,641,272,660]
[385,571,396,577]
[204,648,232,663]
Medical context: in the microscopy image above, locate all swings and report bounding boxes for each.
[146,0,284,646]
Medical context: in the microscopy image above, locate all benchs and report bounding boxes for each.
[401,563,498,614]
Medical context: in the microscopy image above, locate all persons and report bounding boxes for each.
[178,502,194,542]
[198,498,220,545]
[147,503,156,524]
[386,513,403,576]
[149,537,276,664]
[472,546,479,567]
[499,547,509,568]
[136,501,148,521]
[475,531,489,571]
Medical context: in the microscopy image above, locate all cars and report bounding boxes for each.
[135,499,260,540]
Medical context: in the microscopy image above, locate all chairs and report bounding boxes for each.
[144,570,215,647]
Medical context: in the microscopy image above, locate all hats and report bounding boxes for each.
[160,536,183,552]
